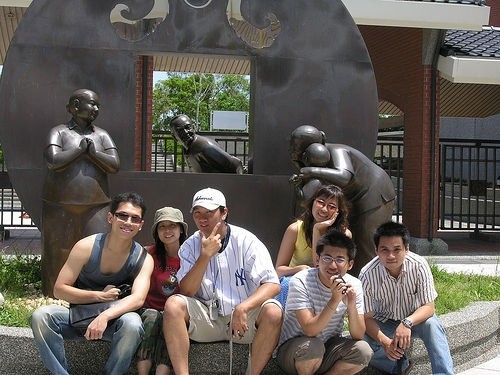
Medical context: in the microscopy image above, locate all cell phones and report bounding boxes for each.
[333,272,348,293]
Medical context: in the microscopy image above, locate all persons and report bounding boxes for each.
[357,222,455,375]
[287,125,395,278]
[42,89,121,300]
[273,184,351,312]
[31,191,155,375]
[271,230,374,375]
[136,206,188,375]
[168,114,245,174]
[162,187,285,375]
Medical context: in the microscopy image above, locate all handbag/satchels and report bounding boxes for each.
[69,284,132,329]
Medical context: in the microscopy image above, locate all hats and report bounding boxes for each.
[151,207,188,239]
[190,188,227,213]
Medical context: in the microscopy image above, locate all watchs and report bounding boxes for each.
[401,318,413,328]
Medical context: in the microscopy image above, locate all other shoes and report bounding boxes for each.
[400,359,415,375]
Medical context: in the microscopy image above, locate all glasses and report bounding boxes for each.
[314,198,338,212]
[114,211,143,224]
[319,254,349,265]
